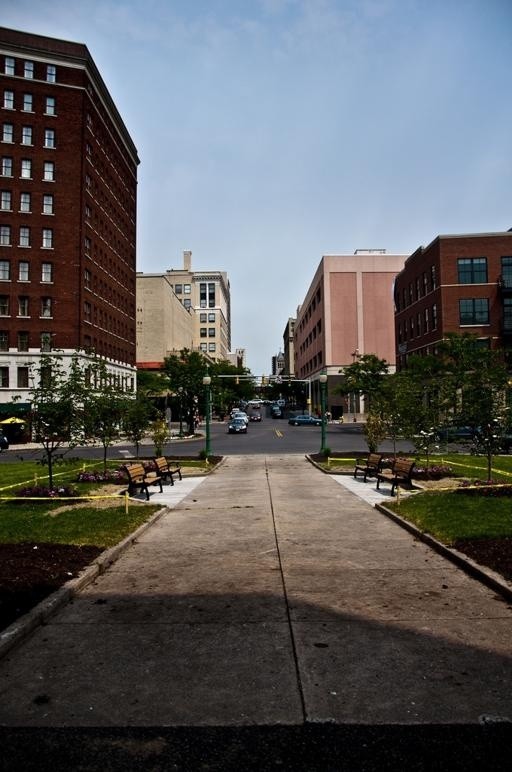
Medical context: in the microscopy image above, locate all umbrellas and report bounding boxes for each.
[0,417,26,425]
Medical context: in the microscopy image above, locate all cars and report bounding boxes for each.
[265,398,286,419]
[228,401,249,434]
[252,403,260,408]
[247,399,264,404]
[250,411,262,421]
[288,415,323,426]
[428,422,477,442]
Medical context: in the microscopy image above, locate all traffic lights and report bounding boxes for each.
[288,379,291,387]
[262,376,266,384]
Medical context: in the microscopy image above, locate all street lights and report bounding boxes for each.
[203,364,212,454]
[320,363,327,449]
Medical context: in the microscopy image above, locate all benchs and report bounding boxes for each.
[152,457,182,486]
[353,453,383,482]
[123,463,163,501]
[376,457,416,497]
